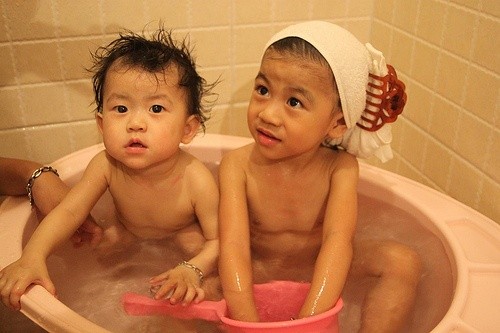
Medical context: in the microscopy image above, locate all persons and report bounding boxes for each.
[200,19,421,323]
[0,21,221,311]
[0,157,104,250]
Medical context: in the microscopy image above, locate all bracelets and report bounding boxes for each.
[25,166,60,211]
[178,261,206,280]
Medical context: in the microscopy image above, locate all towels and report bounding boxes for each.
[261,22,394,164]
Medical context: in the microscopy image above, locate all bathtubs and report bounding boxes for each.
[21,146,457,333]
